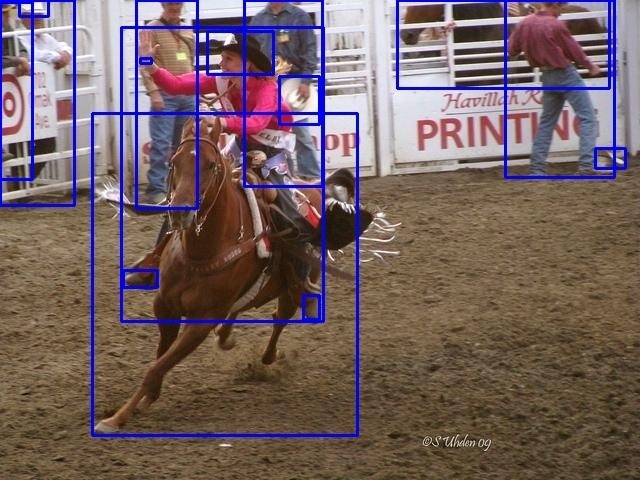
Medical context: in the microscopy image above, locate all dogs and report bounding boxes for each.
[93,113,357,436]
[398,1,610,88]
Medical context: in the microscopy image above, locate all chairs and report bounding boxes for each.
[304,296,319,319]
[123,271,157,287]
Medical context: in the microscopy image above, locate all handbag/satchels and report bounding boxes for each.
[141,58,152,64]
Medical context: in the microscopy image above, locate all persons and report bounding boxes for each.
[137,0,197,208]
[121,28,324,321]
[506,0,615,177]
[245,0,322,187]
[0,0,75,206]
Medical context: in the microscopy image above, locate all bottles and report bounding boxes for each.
[596,148,625,168]
[207,32,274,75]
[280,76,320,124]
[19,1,48,17]
